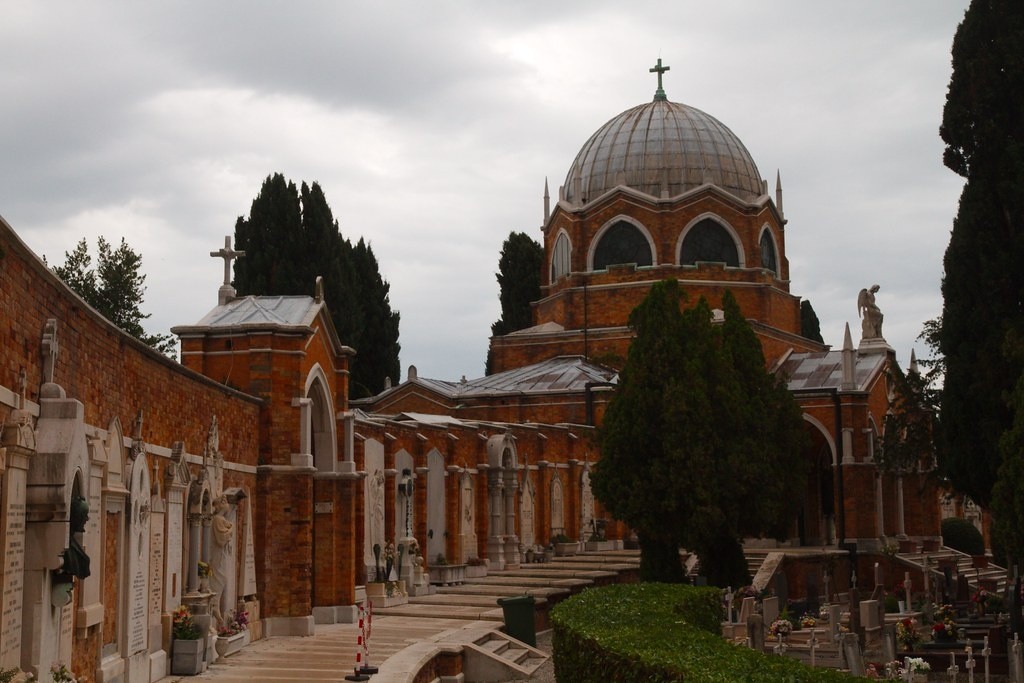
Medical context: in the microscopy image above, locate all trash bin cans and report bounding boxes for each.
[497,595,537,648]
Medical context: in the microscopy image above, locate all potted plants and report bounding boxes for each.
[546,546,553,561]
[465,555,488,577]
[428,553,468,587]
[550,534,578,557]
[585,532,613,551]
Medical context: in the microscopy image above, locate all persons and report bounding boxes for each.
[867,285,883,337]
[209,496,233,624]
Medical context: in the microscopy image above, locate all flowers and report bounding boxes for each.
[216,608,250,638]
[197,561,213,577]
[172,605,200,640]
[725,542,991,683]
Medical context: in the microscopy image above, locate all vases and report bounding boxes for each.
[200,577,209,591]
[976,606,986,617]
[934,634,958,643]
[215,629,250,657]
[903,643,913,652]
[898,600,905,614]
[912,674,928,683]
[173,637,204,674]
